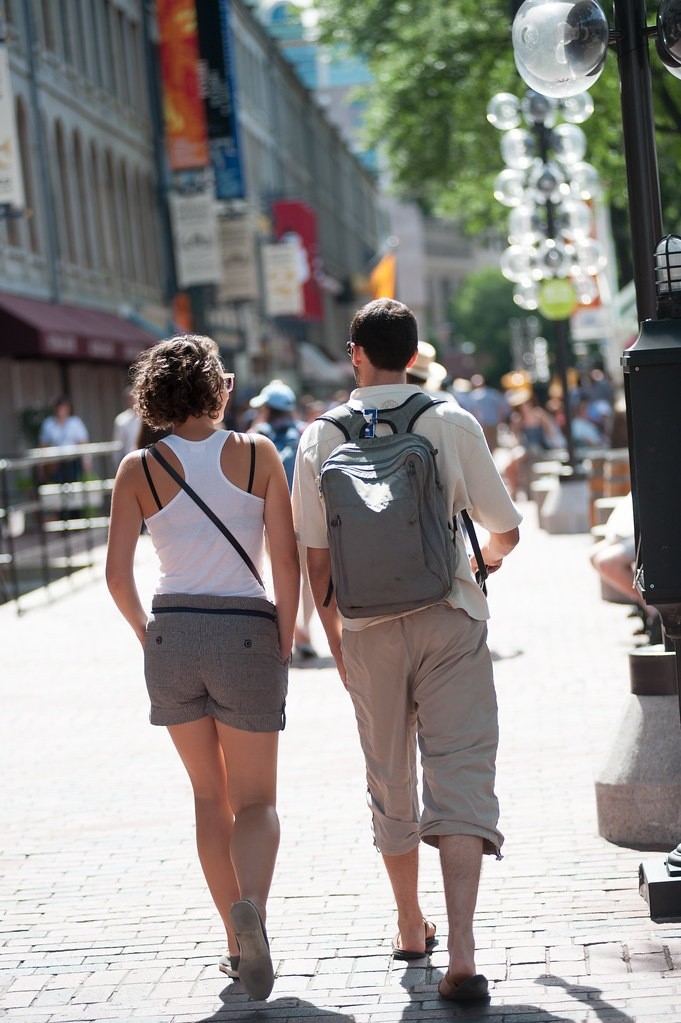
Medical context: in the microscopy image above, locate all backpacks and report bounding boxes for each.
[317,394,456,618]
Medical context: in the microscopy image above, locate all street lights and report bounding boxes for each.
[485,84,609,474]
[504,1,680,336]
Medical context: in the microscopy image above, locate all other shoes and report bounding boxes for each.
[219,954,243,978]
[231,899,273,1001]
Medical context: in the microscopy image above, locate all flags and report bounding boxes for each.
[272,197,324,321]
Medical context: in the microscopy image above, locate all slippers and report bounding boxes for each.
[438,973,488,1000]
[390,915,436,959]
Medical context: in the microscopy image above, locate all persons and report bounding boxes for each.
[37,397,94,537]
[291,295,523,1006]
[304,366,616,502]
[112,386,146,535]
[588,490,647,636]
[249,382,316,655]
[106,333,302,1001]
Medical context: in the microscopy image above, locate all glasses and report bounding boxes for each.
[218,371,234,394]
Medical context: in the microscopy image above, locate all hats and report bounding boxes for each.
[250,383,296,412]
